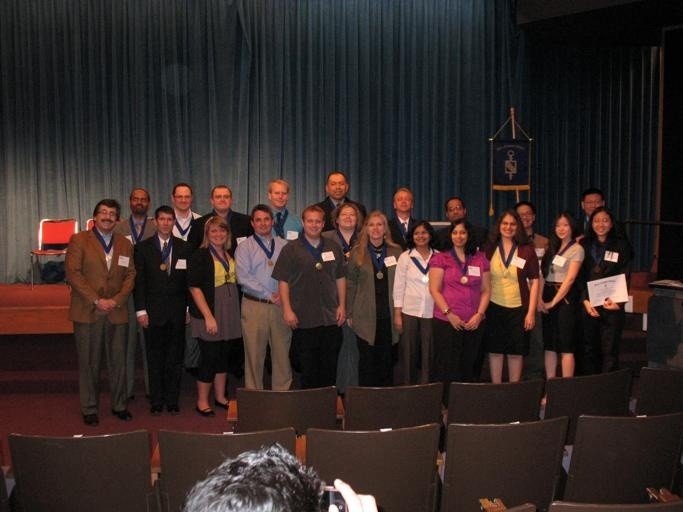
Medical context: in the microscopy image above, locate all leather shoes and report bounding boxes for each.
[112,409,133,422]
[82,413,100,428]
[216,398,230,410]
[195,405,216,417]
[150,403,180,416]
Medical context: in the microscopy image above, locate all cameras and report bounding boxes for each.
[320,485,348,512]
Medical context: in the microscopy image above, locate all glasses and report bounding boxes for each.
[97,210,116,217]
[448,205,463,212]
[585,199,602,205]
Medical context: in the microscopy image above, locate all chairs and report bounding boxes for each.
[31,212,80,286]
[84,217,94,232]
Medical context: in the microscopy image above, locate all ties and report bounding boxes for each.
[337,202,341,208]
[276,212,282,223]
[162,242,169,268]
[136,223,142,238]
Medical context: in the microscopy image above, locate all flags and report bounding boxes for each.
[490,140,531,191]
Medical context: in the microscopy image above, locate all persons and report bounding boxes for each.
[186,442,378,512]
[63,170,632,424]
